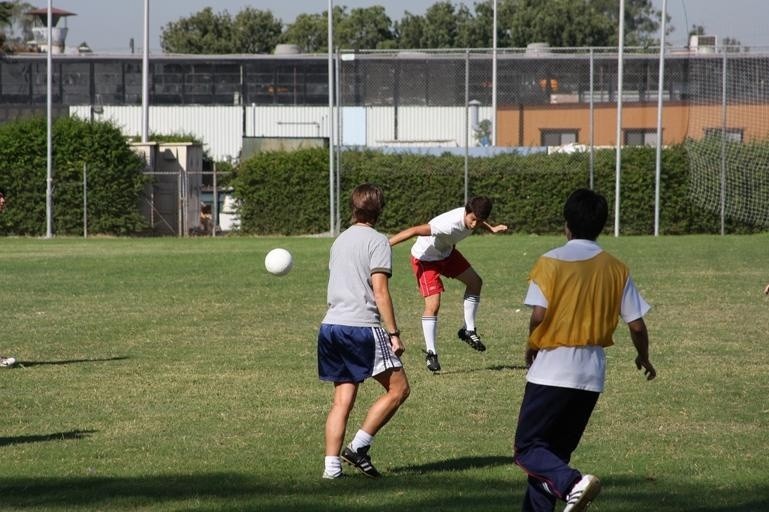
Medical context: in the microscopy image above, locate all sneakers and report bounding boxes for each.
[562,474,601,512]
[457,328,486,352]
[341,444,380,480]
[426,352,441,371]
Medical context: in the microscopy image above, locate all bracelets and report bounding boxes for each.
[387,329,402,339]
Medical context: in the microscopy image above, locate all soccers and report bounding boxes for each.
[264,248,293,276]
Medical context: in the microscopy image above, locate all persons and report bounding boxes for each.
[510,188,657,512]
[387,193,510,368]
[316,182,413,479]
